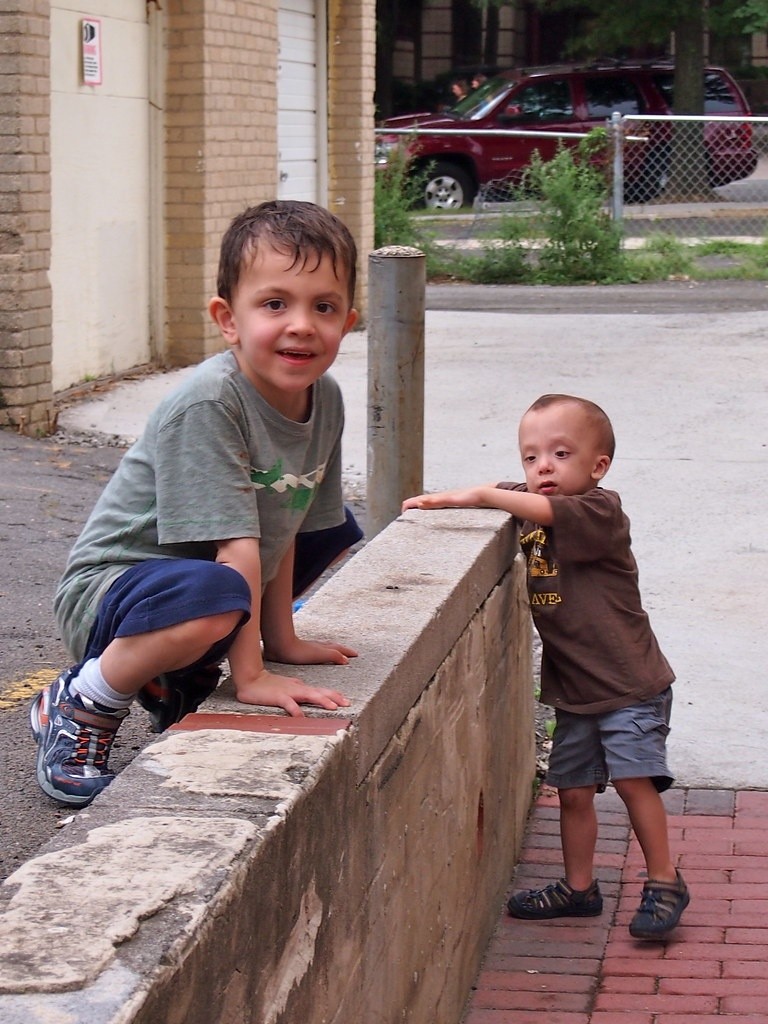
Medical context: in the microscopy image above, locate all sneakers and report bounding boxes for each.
[31,665,130,803]
[141,667,224,731]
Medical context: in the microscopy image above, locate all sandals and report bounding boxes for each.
[629,868,690,937]
[508,877,603,918]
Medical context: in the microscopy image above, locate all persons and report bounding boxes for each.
[452,74,493,113]
[31,202,364,801]
[402,394,689,942]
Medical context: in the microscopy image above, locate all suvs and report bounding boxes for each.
[373,60,759,213]
